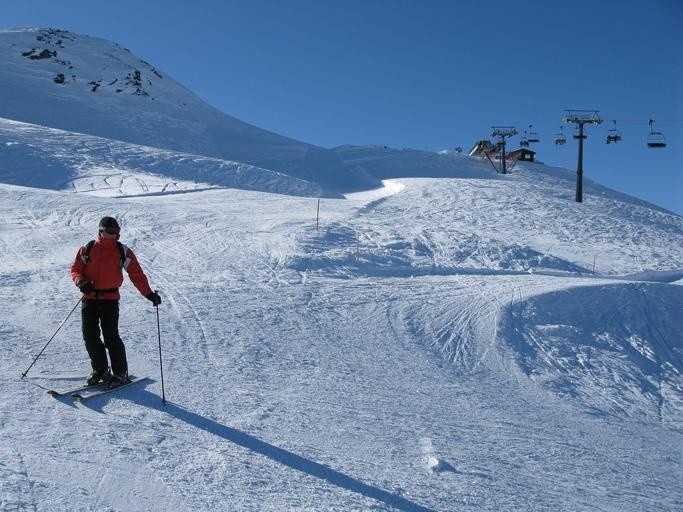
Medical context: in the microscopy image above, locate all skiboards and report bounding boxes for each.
[47,374,148,401]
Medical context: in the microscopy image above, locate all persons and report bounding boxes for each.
[69,216,162,389]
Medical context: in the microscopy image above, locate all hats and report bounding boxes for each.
[99,217,119,228]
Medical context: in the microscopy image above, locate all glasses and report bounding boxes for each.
[105,227,120,235]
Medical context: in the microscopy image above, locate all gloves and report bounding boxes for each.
[146,291,161,306]
[76,279,95,293]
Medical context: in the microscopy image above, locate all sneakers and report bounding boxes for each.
[87,368,110,386]
[106,370,129,390]
